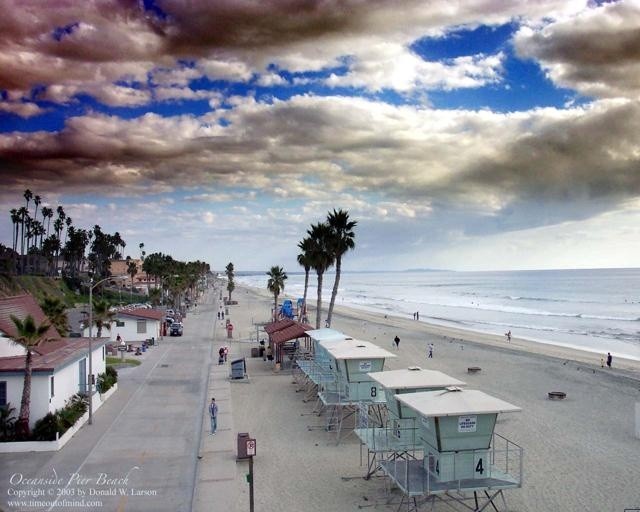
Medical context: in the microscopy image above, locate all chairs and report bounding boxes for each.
[283,342,312,360]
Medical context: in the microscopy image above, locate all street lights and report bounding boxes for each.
[161,274,180,306]
[87,274,128,424]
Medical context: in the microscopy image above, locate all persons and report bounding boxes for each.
[506,330,512,343]
[223,346,229,362]
[217,345,225,365]
[599,357,605,367]
[607,352,613,368]
[266,346,274,365]
[394,335,400,349]
[428,343,434,358]
[116,334,122,347]
[208,397,219,435]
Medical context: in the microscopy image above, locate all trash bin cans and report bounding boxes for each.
[142,344,146,352]
[231,356,246,379]
[237,433,250,459]
[226,319,230,329]
[112,348,117,355]
[223,297,227,305]
[225,308,229,315]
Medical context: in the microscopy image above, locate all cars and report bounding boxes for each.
[110,302,154,312]
[165,308,183,336]
